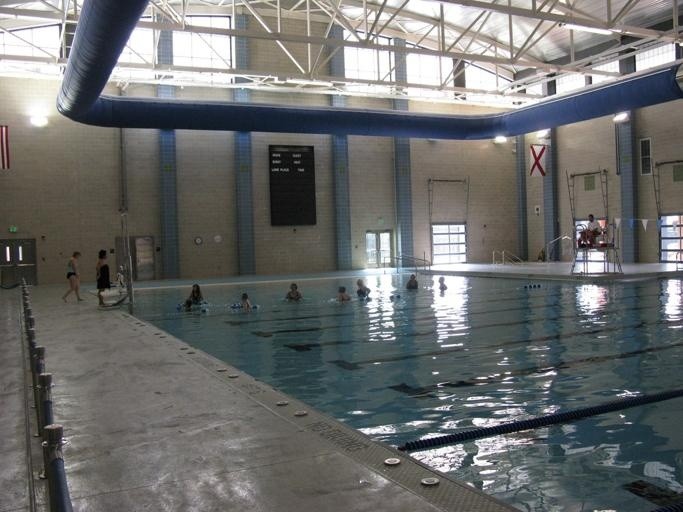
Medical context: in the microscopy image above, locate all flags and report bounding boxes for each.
[528,144,546,176]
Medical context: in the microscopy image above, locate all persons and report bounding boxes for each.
[356,279,370,297]
[580,214,601,245]
[184,300,192,312]
[188,284,203,305]
[94,249,110,306]
[61,252,84,302]
[336,287,350,301]
[437,276,446,289]
[405,274,417,289]
[240,293,251,312]
[284,284,301,301]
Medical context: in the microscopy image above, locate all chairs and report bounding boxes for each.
[592,228,606,243]
